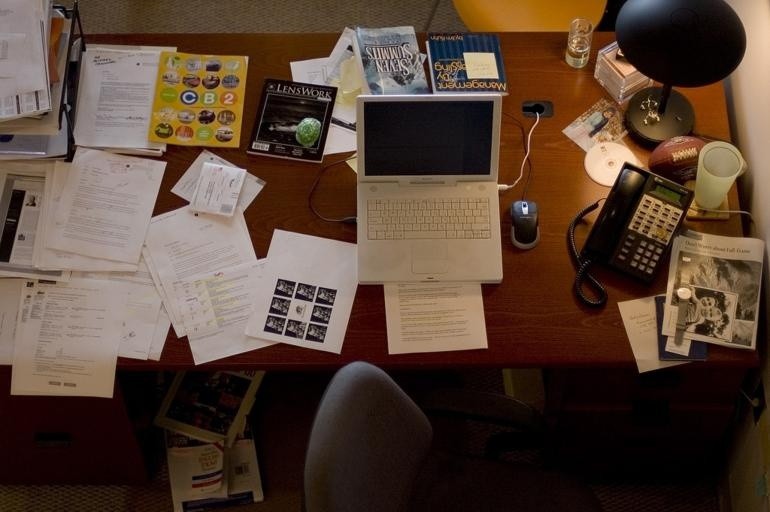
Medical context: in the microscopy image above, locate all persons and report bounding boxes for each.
[565,106,617,151]
[671,301,723,330]
[25,195,36,207]
[669,285,720,330]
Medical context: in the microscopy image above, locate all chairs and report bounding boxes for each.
[303,362,605,512]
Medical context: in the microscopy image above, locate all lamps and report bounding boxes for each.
[614,0,746,151]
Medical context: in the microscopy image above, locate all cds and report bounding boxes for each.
[584,142,637,188]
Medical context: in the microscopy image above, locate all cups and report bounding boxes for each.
[693,140,749,215]
[564,17,594,69]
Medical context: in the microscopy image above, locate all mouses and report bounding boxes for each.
[510,199,539,248]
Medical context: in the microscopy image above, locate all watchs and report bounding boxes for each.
[673,255,693,346]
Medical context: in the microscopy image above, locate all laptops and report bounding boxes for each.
[356,93,505,285]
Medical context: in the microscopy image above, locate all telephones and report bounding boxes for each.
[578,161,694,288]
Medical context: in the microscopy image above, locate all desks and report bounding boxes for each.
[3,30,759,484]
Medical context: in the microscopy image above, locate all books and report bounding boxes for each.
[653,295,709,362]
[153,367,266,451]
[190,440,230,500]
[246,77,338,164]
[425,32,510,96]
[355,25,429,95]
[0,0,179,179]
[661,228,765,350]
[164,438,265,512]
